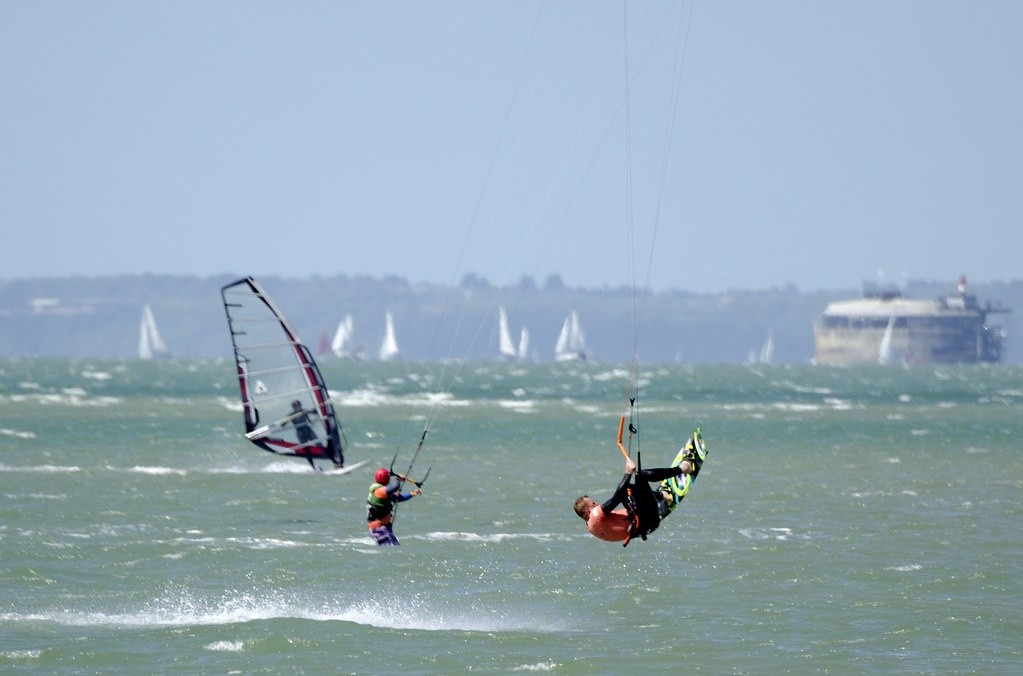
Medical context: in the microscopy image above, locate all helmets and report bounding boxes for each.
[375,468,389,483]
[292,400,301,406]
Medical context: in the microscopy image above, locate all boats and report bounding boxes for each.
[811,273,1015,366]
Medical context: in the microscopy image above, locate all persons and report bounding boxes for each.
[574,450,693,544]
[367,469,424,548]
[280,400,340,470]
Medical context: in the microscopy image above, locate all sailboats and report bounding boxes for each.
[496,304,543,366]
[554,311,593,364]
[221,273,370,478]
[316,313,359,360]
[378,308,404,364]
[138,302,175,360]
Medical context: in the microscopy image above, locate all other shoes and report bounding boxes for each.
[658,478,678,511]
[682,443,698,476]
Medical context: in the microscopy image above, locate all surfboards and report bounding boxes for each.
[637,427,708,537]
